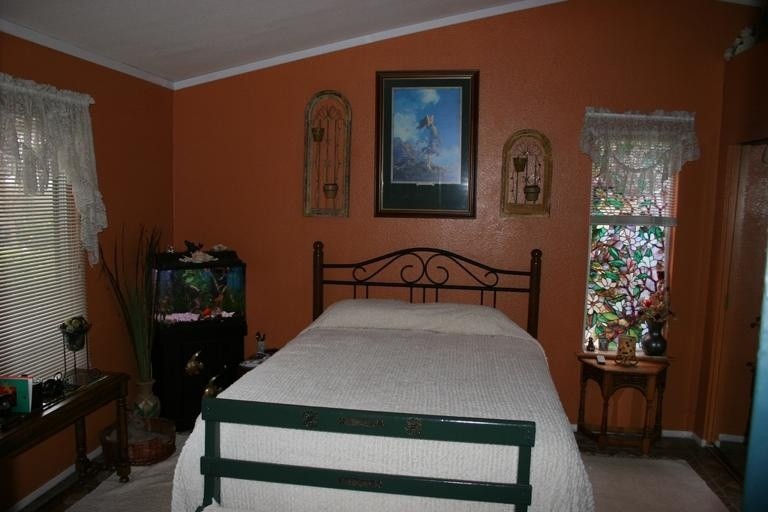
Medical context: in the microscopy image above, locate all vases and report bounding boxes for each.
[641,321,667,354]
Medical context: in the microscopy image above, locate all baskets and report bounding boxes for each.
[98,415,176,467]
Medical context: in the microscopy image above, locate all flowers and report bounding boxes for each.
[639,288,675,323]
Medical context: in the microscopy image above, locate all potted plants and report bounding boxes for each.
[96,223,170,420]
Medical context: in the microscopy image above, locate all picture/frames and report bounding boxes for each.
[374,70,480,220]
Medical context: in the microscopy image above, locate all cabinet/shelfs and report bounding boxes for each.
[152,322,247,433]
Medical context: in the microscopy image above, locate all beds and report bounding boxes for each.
[170,241,596,512]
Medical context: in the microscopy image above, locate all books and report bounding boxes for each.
[0,375,33,414]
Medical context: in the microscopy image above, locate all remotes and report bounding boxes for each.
[596,355,605,363]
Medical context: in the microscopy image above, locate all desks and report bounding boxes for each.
[579,358,668,454]
[1,368,133,512]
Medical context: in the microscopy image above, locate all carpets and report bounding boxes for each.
[66,433,729,512]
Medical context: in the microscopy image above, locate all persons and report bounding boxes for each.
[417,114,440,170]
